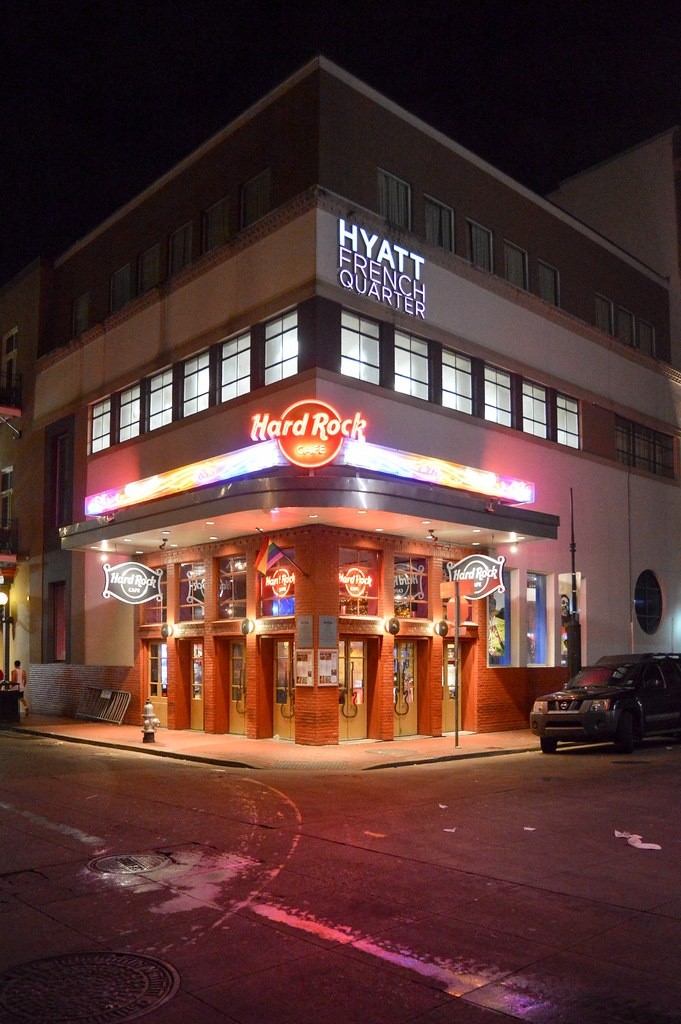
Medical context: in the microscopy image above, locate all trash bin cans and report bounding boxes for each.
[0,680,21,724]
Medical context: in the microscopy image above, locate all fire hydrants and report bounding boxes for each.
[141,699,161,744]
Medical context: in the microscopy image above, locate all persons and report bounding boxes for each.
[10,660,30,716]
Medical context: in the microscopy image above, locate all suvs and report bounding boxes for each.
[529,653,680,755]
